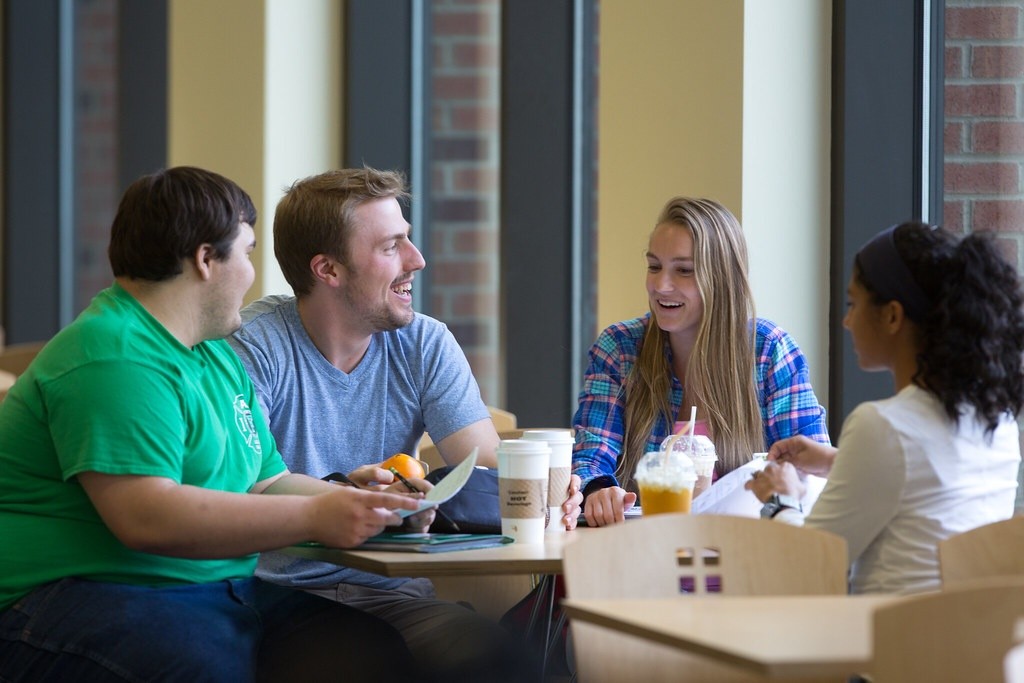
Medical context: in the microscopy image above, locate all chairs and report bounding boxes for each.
[418,406,575,625]
[563,512,848,683]
[851,579,1023,683]
[939,515,1023,591]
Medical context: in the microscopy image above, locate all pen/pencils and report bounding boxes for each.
[388,466,460,531]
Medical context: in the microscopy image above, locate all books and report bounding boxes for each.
[300,530,514,554]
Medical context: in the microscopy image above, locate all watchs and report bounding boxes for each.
[761,494,803,519]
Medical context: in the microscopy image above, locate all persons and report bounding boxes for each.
[225,168,585,683]
[0,168,439,683]
[745,225,1024,595]
[571,198,830,526]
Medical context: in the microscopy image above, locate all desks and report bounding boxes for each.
[264,528,595,683]
[560,594,901,683]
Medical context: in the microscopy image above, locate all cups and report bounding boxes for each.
[497,439,551,544]
[522,428,579,532]
[635,450,695,519]
[660,435,718,501]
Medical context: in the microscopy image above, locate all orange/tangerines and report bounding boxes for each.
[379,453,425,485]
